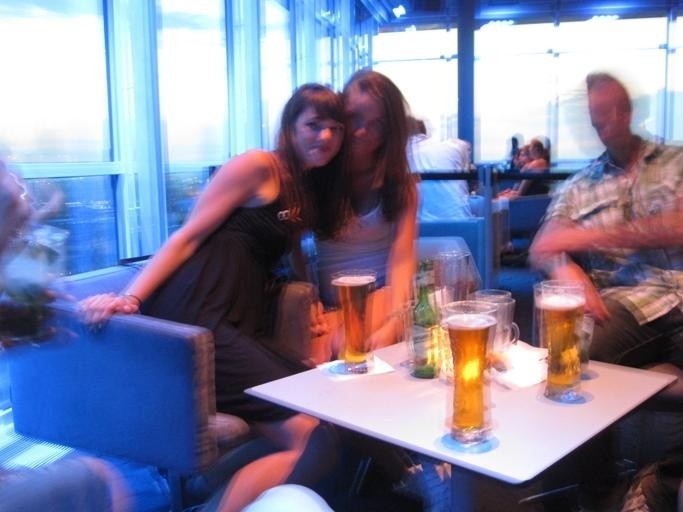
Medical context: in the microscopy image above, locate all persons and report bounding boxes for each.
[0,162,132,511]
[404,114,549,254]
[76,82,347,511]
[314,69,418,485]
[528,70,683,510]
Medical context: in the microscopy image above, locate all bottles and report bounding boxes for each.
[408,258,439,379]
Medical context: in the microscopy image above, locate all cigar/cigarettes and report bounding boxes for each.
[308,147,320,156]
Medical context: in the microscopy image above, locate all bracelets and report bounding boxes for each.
[122,293,141,305]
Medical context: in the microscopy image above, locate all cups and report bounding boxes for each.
[332,275,375,373]
[435,248,519,447]
[533,280,591,402]
